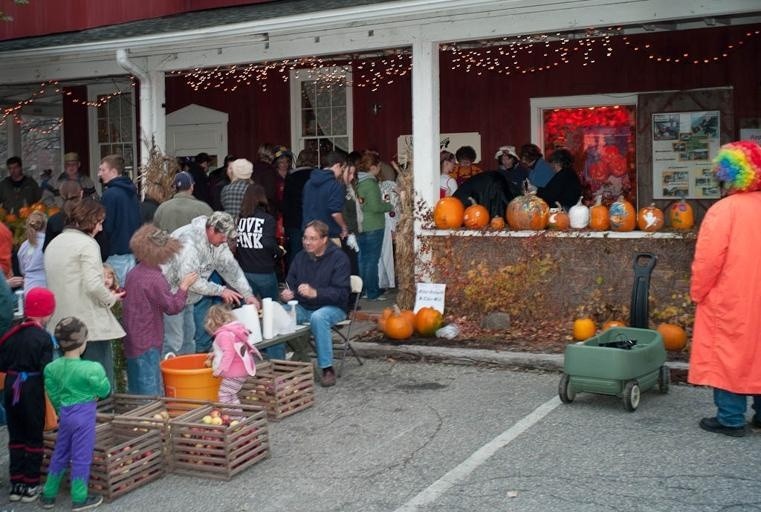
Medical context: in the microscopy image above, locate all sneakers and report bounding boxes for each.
[21,483,41,503]
[8,482,22,502]
[72,494,104,511]
[36,491,56,508]
[320,368,336,387]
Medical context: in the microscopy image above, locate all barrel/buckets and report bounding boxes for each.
[161,351,222,412]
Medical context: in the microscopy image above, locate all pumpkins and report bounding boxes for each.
[601,320,626,333]
[463,197,490,230]
[434,196,464,228]
[491,214,505,231]
[379,303,444,341]
[572,317,595,341]
[505,180,694,231]
[655,323,688,351]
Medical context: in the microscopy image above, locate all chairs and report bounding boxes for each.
[300,274,363,379]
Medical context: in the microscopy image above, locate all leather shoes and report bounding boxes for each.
[699,411,761,437]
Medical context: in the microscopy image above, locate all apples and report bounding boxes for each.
[88,380,313,497]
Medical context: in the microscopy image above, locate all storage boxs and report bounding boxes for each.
[169,402,272,482]
[38,414,110,487]
[113,397,212,474]
[95,393,160,423]
[87,421,163,503]
[237,358,316,423]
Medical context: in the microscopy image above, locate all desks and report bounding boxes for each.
[249,302,320,383]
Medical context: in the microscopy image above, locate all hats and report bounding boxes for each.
[230,157,254,180]
[170,171,198,189]
[272,144,293,164]
[63,152,81,163]
[207,209,239,240]
[54,316,88,351]
[494,145,522,162]
[23,286,57,319]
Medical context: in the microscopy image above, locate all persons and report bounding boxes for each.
[686,139,761,437]
[0,141,126,511]
[95,150,417,424]
[440,141,584,228]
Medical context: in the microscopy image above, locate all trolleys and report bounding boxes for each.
[555,250,676,412]
